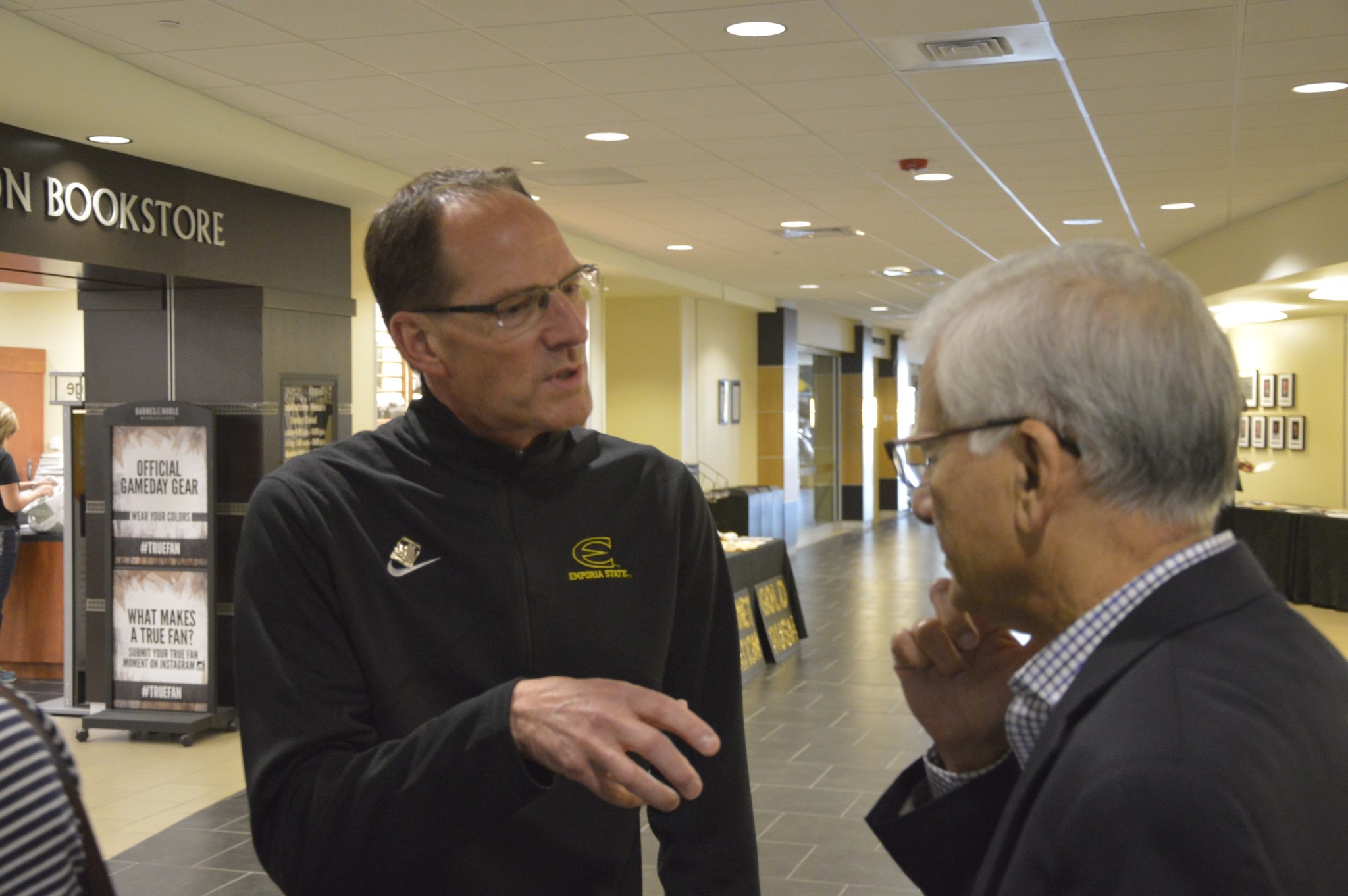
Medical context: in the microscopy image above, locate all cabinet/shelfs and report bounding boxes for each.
[1,346,66,682]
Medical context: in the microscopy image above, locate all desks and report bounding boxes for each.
[1226,498,1348,615]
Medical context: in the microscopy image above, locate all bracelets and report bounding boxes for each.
[30,480,35,490]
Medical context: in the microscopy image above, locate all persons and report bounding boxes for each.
[0,402,60,684]
[228,161,766,896]
[862,241,1348,896]
[0,690,108,896]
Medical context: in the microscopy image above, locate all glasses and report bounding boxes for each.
[402,264,599,329]
[884,415,1081,489]
